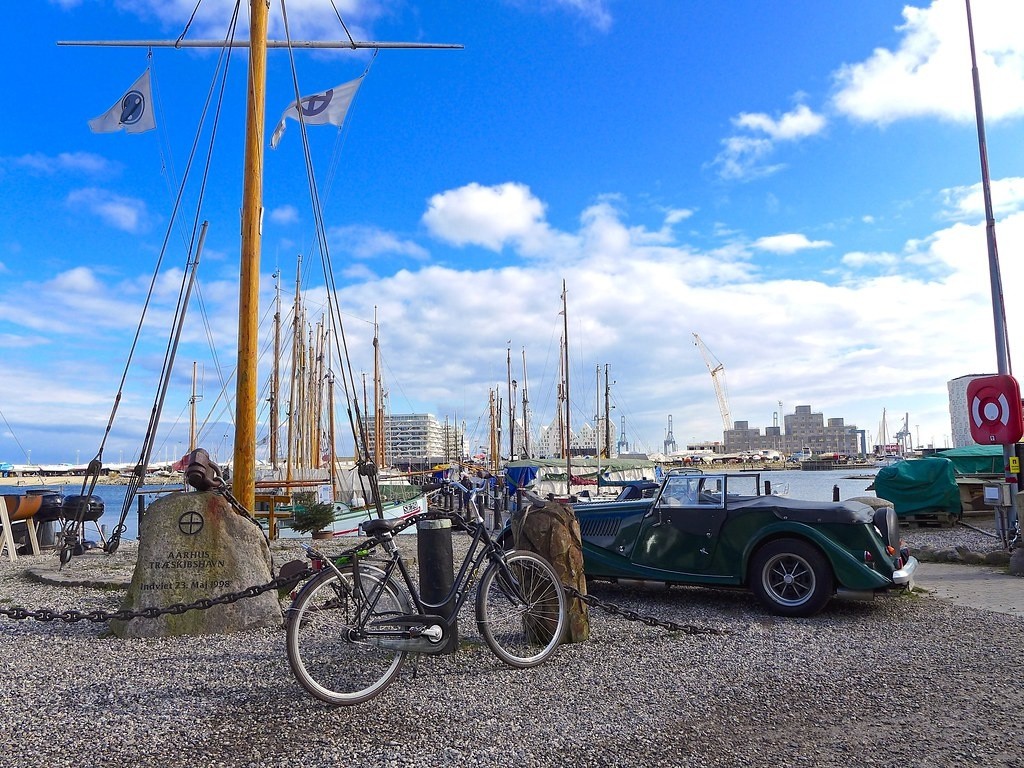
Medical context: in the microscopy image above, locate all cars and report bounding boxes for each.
[491,466,919,620]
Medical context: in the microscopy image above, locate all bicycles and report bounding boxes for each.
[282,471,567,707]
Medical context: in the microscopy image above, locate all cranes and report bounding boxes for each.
[617,415,629,455]
[691,333,734,431]
[663,414,676,456]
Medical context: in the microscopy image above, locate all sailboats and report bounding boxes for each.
[65,0,663,541]
